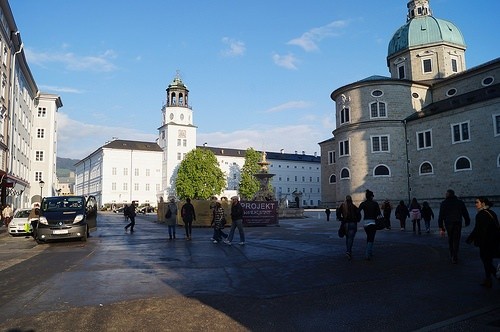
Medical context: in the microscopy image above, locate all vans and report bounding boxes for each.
[30,195,98,244]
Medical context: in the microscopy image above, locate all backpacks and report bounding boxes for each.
[125,207,130,215]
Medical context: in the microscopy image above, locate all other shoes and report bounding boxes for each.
[189,236,191,240]
[224,241,231,245]
[238,241,245,245]
[213,240,218,244]
[210,238,215,240]
[346,252,351,258]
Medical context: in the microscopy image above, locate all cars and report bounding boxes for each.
[137,206,155,213]
[112,207,124,214]
[7,208,32,237]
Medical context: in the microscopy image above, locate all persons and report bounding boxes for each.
[122,200,153,235]
[210,196,245,245]
[469,196,499,290]
[0,203,40,240]
[181,198,197,240]
[165,197,178,240]
[326,189,471,267]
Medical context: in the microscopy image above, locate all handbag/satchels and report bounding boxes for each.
[221,219,227,226]
[375,213,387,230]
[339,222,346,237]
[440,228,445,240]
[165,205,171,219]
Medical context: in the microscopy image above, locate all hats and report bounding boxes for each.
[170,199,175,202]
[231,196,238,200]
[366,189,374,199]
[216,200,222,204]
[6,204,10,207]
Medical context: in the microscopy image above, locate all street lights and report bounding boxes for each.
[39,181,46,201]
[56,188,61,196]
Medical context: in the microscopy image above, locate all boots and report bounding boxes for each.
[169,233,172,239]
[173,231,175,239]
[480,277,492,288]
